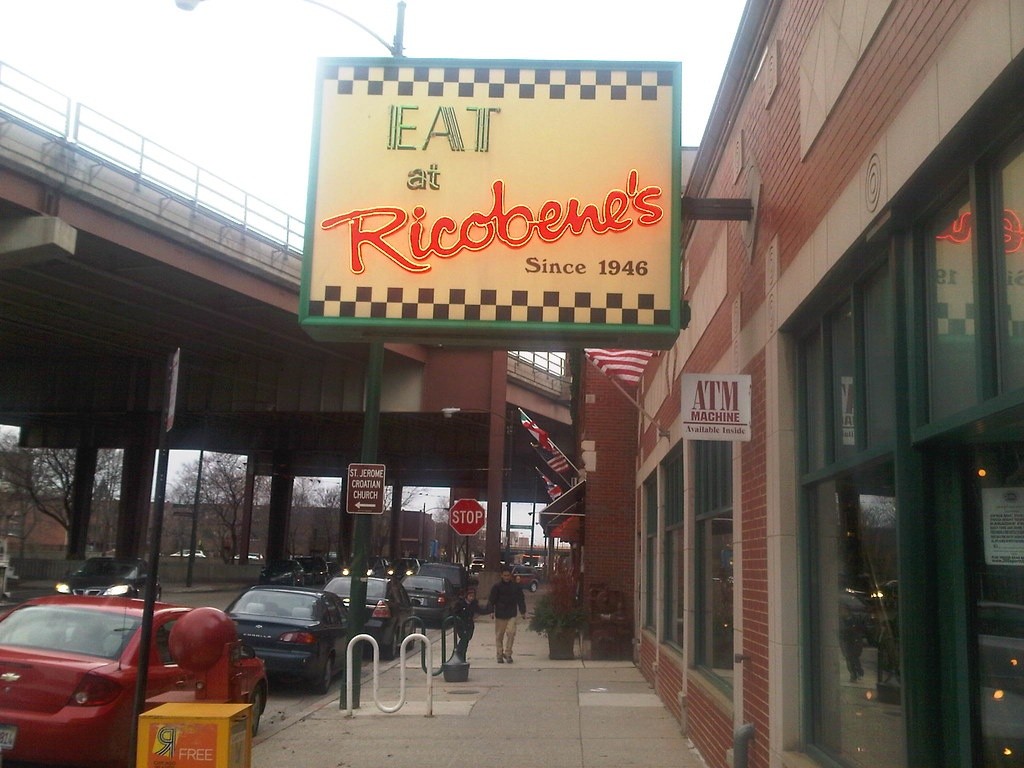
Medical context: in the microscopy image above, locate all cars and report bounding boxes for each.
[260,551,548,629]
[322,576,417,656]
[55,557,162,602]
[0,594,268,768]
[222,585,349,694]
[235,552,263,560]
[834,578,899,646]
[170,550,206,558]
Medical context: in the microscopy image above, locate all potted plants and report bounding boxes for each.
[525,590,589,661]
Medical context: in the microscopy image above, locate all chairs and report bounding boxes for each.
[246,602,266,613]
[290,607,312,617]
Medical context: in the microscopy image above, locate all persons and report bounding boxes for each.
[487,567,527,664]
[452,589,492,663]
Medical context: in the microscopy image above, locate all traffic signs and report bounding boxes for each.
[346,463,386,515]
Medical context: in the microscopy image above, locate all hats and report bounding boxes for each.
[466,587,476,595]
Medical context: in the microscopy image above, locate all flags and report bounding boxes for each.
[521,413,554,451]
[584,348,655,387]
[532,443,571,474]
[537,471,562,504]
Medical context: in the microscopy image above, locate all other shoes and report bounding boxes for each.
[455,649,467,663]
[498,658,504,663]
[503,653,513,663]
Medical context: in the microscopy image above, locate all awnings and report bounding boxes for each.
[539,479,586,543]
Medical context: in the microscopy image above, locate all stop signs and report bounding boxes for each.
[449,499,486,536]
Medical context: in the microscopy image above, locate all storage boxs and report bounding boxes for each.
[135,703,253,768]
